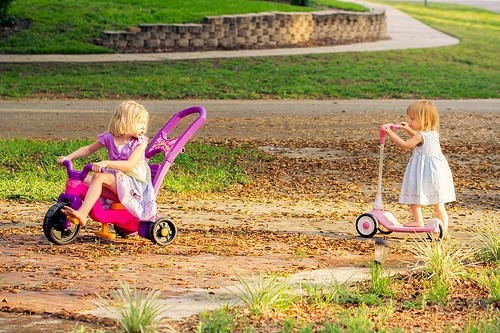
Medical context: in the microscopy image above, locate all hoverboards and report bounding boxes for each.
[353,123,444,242]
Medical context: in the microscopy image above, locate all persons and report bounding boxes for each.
[383,100,456,239]
[55,100,158,226]
